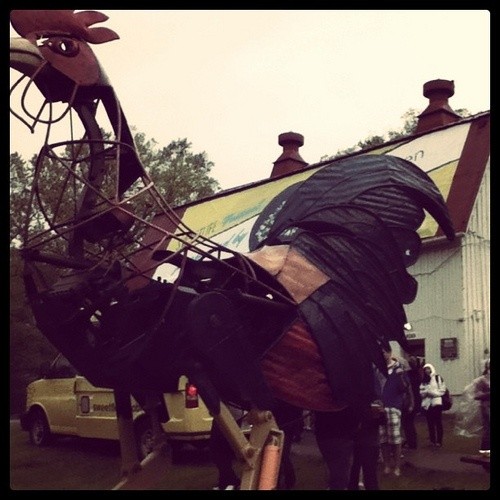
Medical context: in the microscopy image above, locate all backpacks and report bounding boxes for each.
[436,374,452,409]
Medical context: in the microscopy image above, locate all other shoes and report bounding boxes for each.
[406,443,418,450]
[427,443,435,448]
[434,442,441,447]
[479,449,489,457]
[225,485,234,490]
[383,466,390,474]
[394,468,400,477]
[213,485,221,490]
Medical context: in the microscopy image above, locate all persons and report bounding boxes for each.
[255,398,307,489]
[206,396,244,490]
[472,358,490,454]
[417,362,446,446]
[308,336,421,491]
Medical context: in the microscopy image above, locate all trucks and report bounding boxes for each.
[21,351,252,447]
